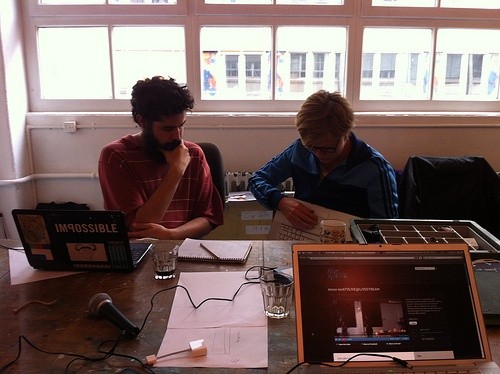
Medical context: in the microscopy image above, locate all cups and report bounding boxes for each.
[151,243,178,280]
[319,219,347,246]
[260,273,294,319]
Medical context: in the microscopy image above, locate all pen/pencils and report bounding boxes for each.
[200,243,220,260]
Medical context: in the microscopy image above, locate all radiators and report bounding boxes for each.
[225,171,294,195]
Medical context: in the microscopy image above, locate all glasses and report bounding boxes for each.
[305,136,342,154]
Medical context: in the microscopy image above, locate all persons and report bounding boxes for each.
[248,92,398,231]
[99,76,224,240]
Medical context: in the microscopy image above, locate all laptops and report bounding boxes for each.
[290,241,500,374]
[267,198,362,244]
[10,208,155,273]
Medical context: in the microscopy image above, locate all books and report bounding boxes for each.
[172,238,252,264]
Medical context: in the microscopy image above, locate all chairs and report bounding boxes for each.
[196,143,225,210]
[396,156,500,240]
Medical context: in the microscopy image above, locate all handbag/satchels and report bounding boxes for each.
[401,155,500,221]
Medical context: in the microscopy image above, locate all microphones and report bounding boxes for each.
[88,292,140,340]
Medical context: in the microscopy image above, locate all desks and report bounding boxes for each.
[202,190,295,240]
[0,239,500,374]
